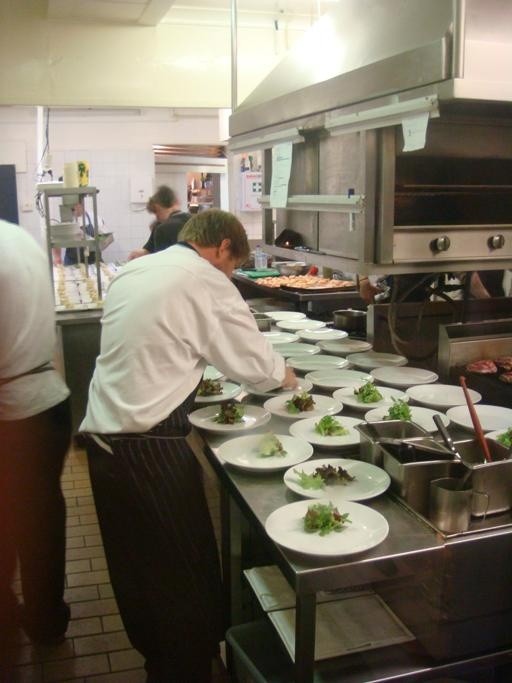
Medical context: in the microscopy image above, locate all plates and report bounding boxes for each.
[445,399,512,432]
[243,375,312,397]
[187,403,271,431]
[263,392,343,418]
[315,339,371,358]
[216,433,314,469]
[403,380,484,408]
[193,380,240,403]
[363,405,450,434]
[285,415,369,448]
[54,262,119,305]
[330,385,410,411]
[283,459,392,501]
[263,309,307,320]
[368,366,440,390]
[295,327,350,344]
[50,222,81,240]
[286,355,349,371]
[271,341,321,356]
[260,330,298,343]
[345,352,410,369]
[264,499,389,560]
[303,369,375,389]
[274,318,325,331]
[37,182,64,188]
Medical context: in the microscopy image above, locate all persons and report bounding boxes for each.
[0,219,70,648]
[153,186,193,252]
[53,202,105,265]
[128,197,161,260]
[77,209,296,683]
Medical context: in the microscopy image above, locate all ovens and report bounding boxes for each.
[376,122,512,264]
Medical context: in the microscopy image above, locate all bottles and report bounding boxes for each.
[256,245,260,256]
[63,157,80,188]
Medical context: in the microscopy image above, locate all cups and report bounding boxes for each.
[428,476,491,534]
[255,253,267,270]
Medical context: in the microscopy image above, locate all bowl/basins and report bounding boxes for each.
[275,262,311,274]
